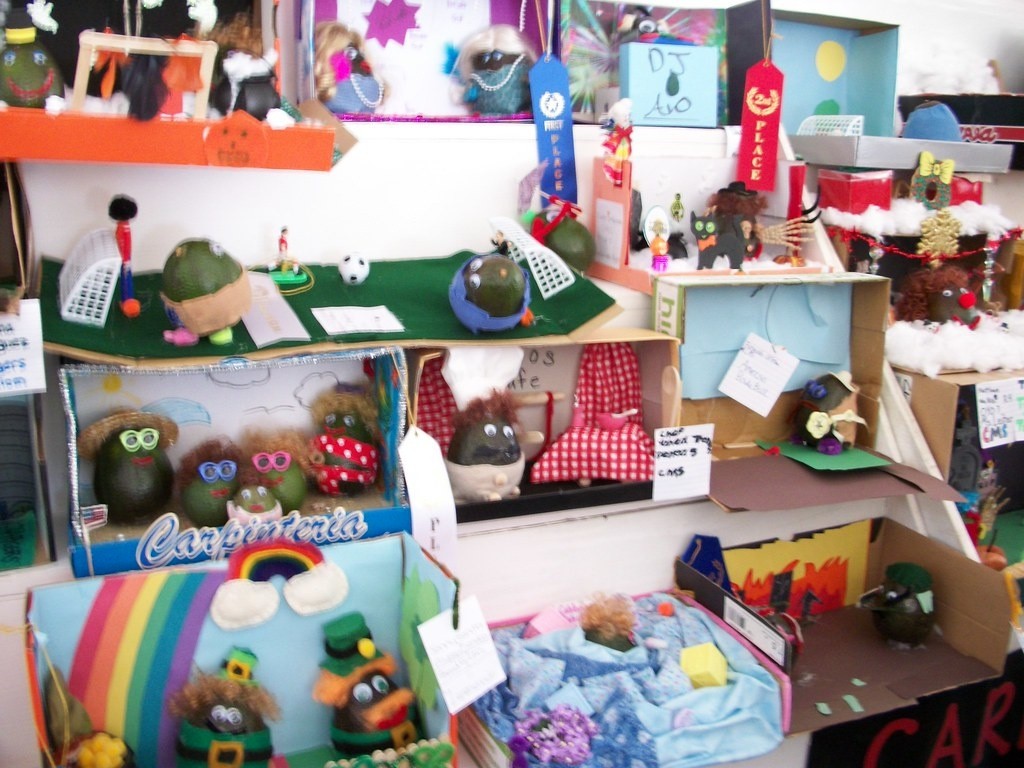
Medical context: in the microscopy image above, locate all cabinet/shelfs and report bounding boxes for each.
[0,120,1024,768]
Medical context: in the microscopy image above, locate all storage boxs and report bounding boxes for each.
[897,92,1024,173]
[292,0,549,124]
[619,42,719,129]
[554,0,773,129]
[771,8,901,137]
[586,155,834,297]
[674,517,1010,736]
[787,132,1014,175]
[0,106,357,172]
[459,588,793,768]
[25,533,462,768]
[649,271,893,514]
[54,347,413,579]
[889,362,1024,485]
[409,327,682,525]
